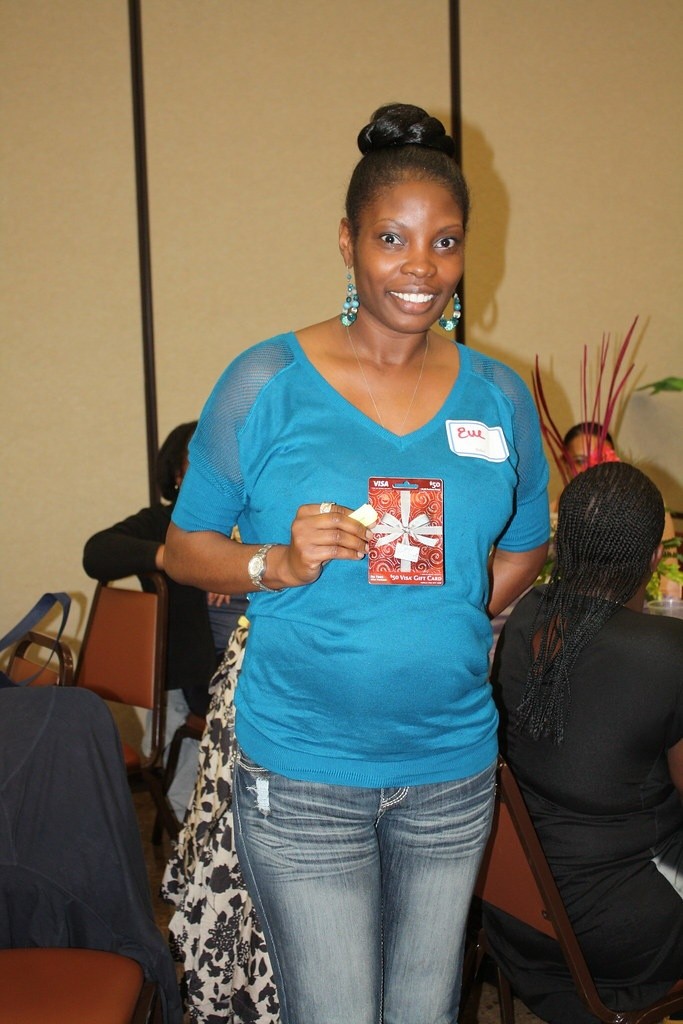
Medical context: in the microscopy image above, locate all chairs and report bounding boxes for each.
[6,626,74,687]
[67,568,206,848]
[0,684,188,1024]
[458,751,683,1024]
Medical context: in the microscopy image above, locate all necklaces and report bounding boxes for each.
[345,322,429,433]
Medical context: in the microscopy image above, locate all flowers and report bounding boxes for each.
[522,312,683,613]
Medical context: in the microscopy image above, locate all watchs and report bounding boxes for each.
[248,544,284,593]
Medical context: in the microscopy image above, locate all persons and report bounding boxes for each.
[82,419,252,866]
[490,461,683,1024]
[163,103,556,1024]
[549,421,674,544]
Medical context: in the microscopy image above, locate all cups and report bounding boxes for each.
[647,599,683,619]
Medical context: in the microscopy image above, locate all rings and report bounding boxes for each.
[319,502,336,514]
